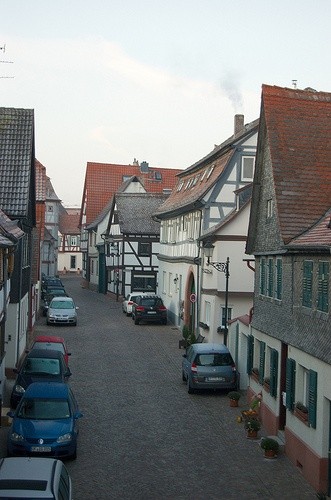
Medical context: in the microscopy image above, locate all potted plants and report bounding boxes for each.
[244,418,262,438]
[228,391,240,407]
[179,325,190,348]
[261,437,278,457]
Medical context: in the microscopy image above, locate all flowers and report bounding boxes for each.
[248,391,262,412]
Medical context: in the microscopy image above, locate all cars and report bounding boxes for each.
[181,342,237,393]
[41,272,80,325]
[121,292,168,325]
[6,334,83,462]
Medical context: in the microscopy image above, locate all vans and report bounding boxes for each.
[0,457,73,500]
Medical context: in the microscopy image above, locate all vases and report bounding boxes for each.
[240,411,257,422]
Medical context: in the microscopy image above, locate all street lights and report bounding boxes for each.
[47,236,56,276]
[204,240,231,346]
[107,233,120,302]
